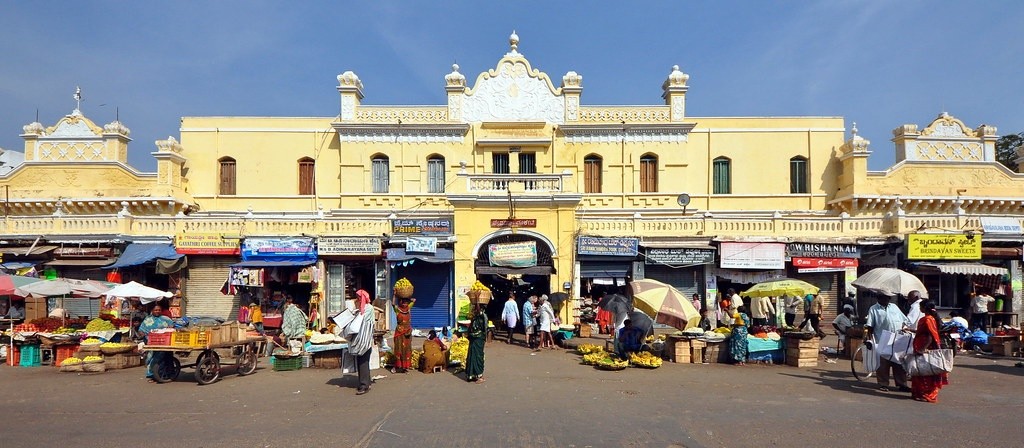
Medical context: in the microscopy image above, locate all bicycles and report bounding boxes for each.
[850,320,879,379]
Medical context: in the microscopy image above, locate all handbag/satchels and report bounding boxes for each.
[876,329,914,365]
[899,348,953,377]
[860,340,881,372]
[551,322,559,332]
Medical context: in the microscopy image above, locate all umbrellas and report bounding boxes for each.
[548,292,570,307]
[850,266,931,302]
[630,312,655,336]
[598,294,631,315]
[627,278,702,354]
[739,276,822,340]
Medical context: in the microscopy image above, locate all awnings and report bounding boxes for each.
[0,242,319,268]
[385,247,456,265]
[913,260,1009,279]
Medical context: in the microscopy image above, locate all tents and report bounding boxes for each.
[0,273,173,367]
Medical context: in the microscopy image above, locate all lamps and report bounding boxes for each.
[677,194,691,215]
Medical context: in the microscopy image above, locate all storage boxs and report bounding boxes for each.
[784,357,818,369]
[666,332,706,363]
[787,335,820,349]
[147,331,173,346]
[273,358,301,371]
[392,286,413,299]
[170,329,212,349]
[466,289,491,305]
[374,306,388,331]
[0,326,141,370]
[786,346,820,359]
[846,325,865,338]
[272,350,291,360]
[579,324,591,338]
[213,319,248,345]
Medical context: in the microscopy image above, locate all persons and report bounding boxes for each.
[137,305,175,378]
[501,291,521,343]
[284,296,295,314]
[438,326,453,344]
[346,289,377,394]
[617,319,649,359]
[692,283,995,368]
[864,289,913,392]
[422,329,447,373]
[326,316,337,334]
[910,299,950,403]
[523,294,558,353]
[464,289,487,383]
[391,289,417,374]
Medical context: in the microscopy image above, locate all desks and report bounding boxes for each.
[987,311,1019,330]
[987,335,1020,357]
[746,337,785,363]
[306,342,350,370]
[261,313,284,332]
[850,337,864,362]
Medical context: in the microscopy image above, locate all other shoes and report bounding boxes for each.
[898,386,911,392]
[507,336,557,352]
[358,385,372,390]
[882,386,890,391]
[356,388,369,395]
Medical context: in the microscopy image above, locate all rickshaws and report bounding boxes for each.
[140,325,267,383]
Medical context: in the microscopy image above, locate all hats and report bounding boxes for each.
[877,286,897,297]
